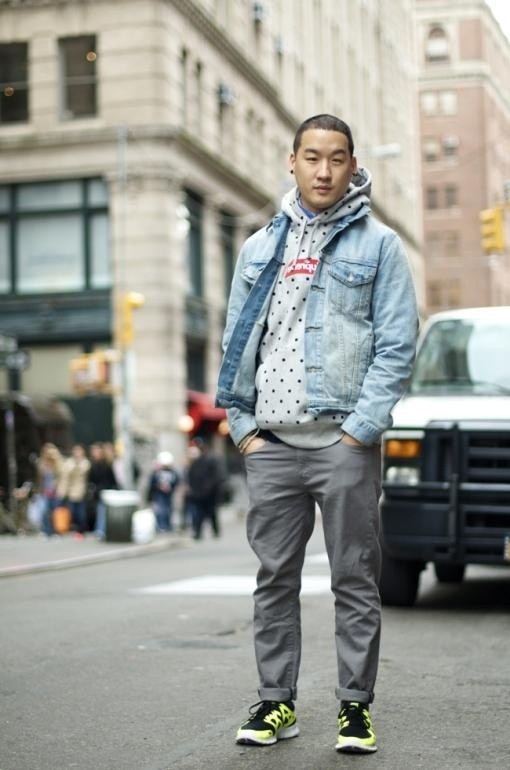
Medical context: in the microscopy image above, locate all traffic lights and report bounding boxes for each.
[113,288,145,346]
[477,206,507,256]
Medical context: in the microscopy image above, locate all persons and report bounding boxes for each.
[213,112,421,757]
[2,438,224,541]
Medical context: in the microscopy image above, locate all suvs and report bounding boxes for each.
[376,305,510,606]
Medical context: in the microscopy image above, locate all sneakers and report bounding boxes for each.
[235,699,299,747]
[336,700,377,755]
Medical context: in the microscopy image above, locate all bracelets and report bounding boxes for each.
[240,427,260,454]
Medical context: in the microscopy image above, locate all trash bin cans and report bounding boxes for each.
[100,489,142,542]
